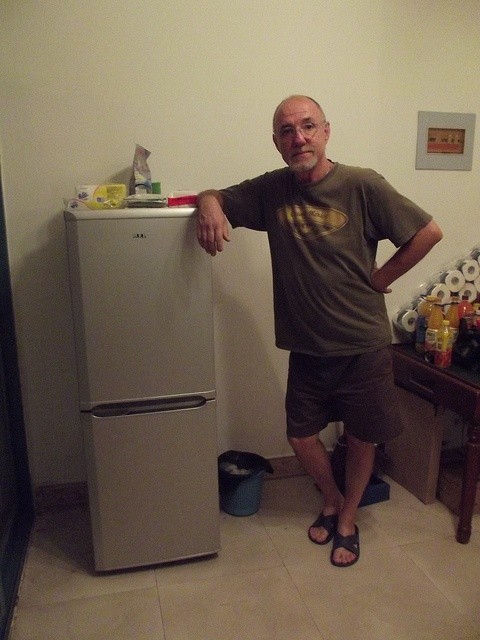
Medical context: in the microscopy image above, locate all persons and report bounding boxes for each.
[197,96,444,568]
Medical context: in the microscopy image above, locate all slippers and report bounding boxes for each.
[330,523,360,568]
[308,510,339,545]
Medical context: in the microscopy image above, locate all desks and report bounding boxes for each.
[387,342,480,544]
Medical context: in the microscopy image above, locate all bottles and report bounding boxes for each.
[473,310,479,328]
[461,296,472,314]
[425,305,444,359]
[436,321,452,365]
[446,291,459,323]
[443,303,459,340]
[415,296,430,352]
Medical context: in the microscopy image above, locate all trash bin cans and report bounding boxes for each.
[218,450,274,517]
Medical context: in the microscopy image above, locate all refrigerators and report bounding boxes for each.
[63,206,222,578]
